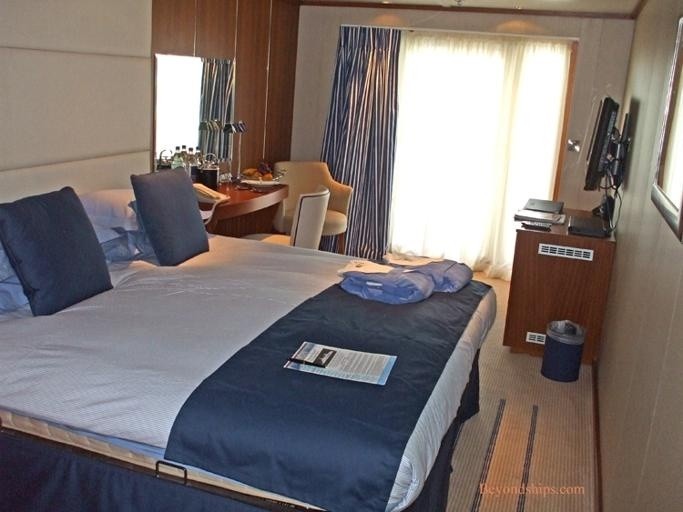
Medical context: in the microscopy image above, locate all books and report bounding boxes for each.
[515,208,566,225]
[513,197,567,213]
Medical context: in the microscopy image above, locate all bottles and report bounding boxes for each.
[171,145,204,169]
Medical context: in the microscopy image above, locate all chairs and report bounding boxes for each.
[273,161,354,254]
[239,185,331,250]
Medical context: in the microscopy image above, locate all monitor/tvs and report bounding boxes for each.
[576,91,619,191]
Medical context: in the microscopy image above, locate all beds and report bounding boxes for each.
[0,230,497,512]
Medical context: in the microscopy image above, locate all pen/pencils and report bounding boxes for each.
[287,357,325,368]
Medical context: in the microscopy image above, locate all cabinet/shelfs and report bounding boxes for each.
[198,177,289,237]
[502,207,617,365]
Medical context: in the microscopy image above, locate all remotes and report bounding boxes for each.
[521,220,551,230]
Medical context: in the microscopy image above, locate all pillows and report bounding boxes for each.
[77,190,213,235]
[0,186,114,316]
[129,166,209,266]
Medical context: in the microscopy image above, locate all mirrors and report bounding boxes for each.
[153,53,236,183]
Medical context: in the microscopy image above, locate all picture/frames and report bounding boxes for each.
[652,19,682,243]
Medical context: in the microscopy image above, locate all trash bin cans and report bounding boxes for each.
[540,319,588,382]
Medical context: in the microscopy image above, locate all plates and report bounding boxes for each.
[241,180,281,188]
